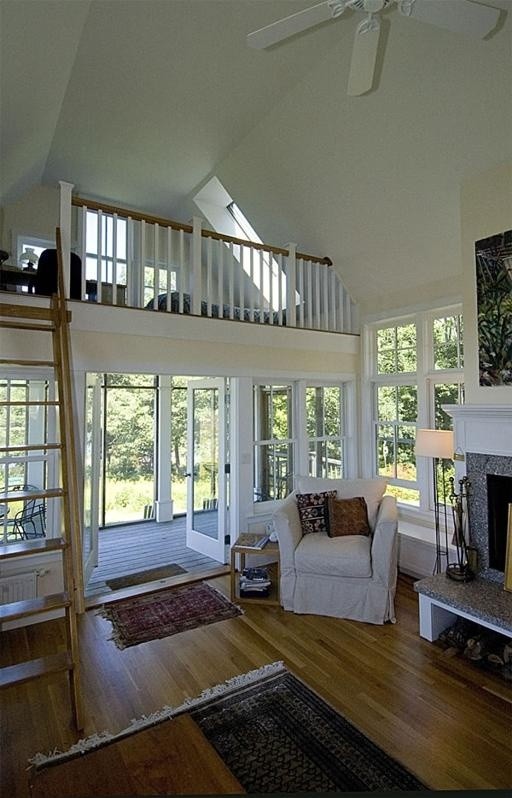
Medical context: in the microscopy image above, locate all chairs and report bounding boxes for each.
[270,473,406,627]
[0,483,48,541]
[36,248,82,299]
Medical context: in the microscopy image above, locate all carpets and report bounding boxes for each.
[92,580,247,651]
[26,660,433,793]
[104,562,188,592]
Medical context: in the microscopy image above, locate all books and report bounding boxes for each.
[239,568,272,597]
[234,532,270,550]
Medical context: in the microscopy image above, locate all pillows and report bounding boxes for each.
[292,490,340,536]
[322,494,372,537]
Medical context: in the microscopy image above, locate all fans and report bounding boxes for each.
[242,1,505,100]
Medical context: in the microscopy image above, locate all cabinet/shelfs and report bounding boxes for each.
[90,278,128,306]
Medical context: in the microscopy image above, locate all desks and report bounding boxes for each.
[229,531,283,609]
[0,266,99,302]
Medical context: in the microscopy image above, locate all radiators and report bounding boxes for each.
[0,567,48,605]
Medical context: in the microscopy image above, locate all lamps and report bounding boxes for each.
[410,428,455,579]
[21,248,38,271]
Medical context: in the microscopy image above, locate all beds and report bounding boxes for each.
[146,292,305,327]
